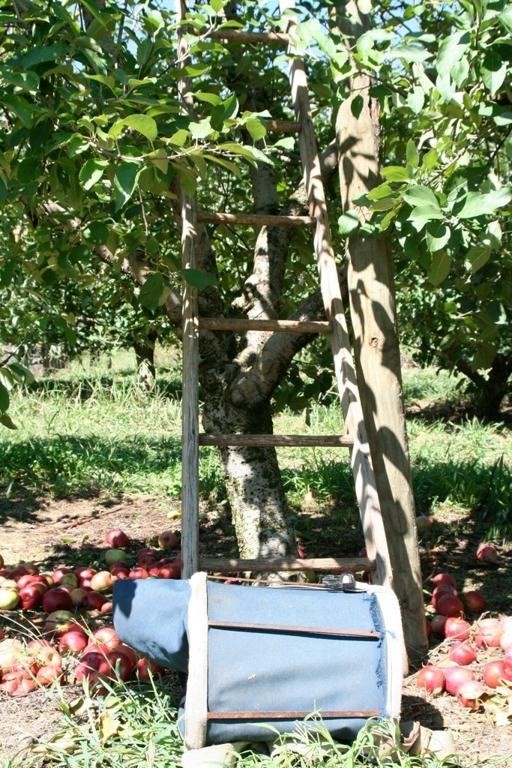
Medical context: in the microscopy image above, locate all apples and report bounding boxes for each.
[0,531,181,698]
[416,517,433,532]
[477,543,498,562]
[416,573,512,709]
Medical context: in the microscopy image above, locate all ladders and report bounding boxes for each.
[177,0,411,677]
